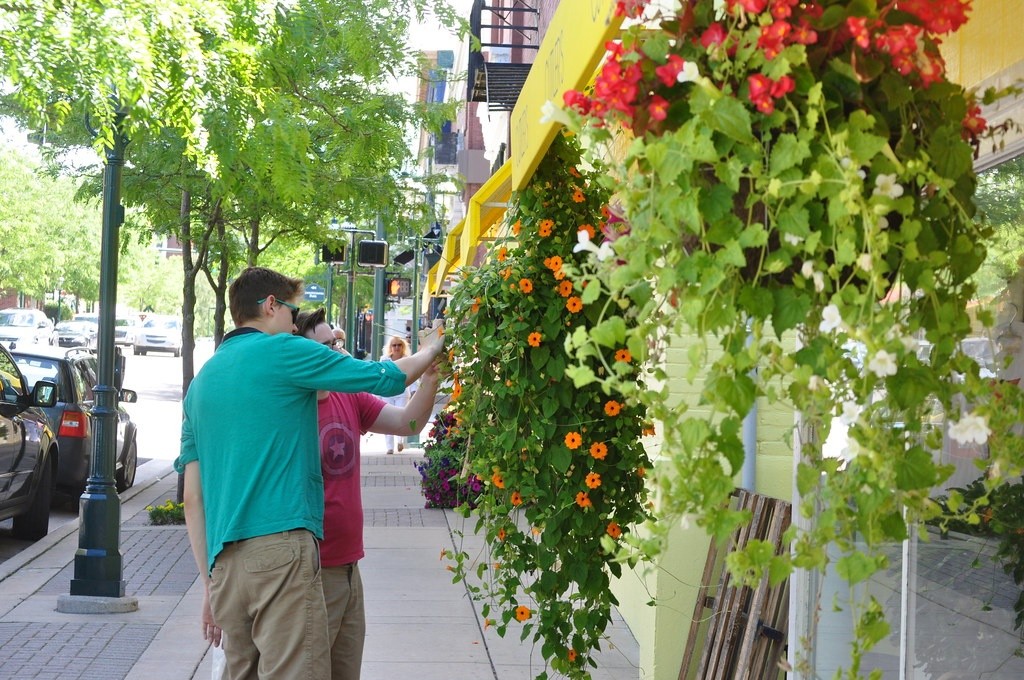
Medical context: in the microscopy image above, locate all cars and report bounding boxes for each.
[72,313,99,324]
[0,342,137,507]
[115,317,135,348]
[0,343,61,541]
[0,307,60,346]
[53,320,98,348]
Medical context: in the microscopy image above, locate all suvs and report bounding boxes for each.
[134,316,182,357]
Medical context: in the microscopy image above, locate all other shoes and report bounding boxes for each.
[398,443,403,452]
[387,449,394,454]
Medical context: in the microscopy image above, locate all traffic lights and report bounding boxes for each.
[390,279,412,298]
[365,313,373,323]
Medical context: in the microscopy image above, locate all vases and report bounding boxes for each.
[677,156,915,299]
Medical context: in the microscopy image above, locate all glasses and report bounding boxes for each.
[258,297,300,324]
[321,338,346,351]
[391,343,403,347]
[406,336,411,338]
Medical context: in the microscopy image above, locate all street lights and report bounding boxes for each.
[69,11,144,599]
[57,275,66,321]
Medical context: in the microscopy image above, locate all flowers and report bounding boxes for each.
[546,0,1024,680]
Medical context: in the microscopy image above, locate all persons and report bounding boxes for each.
[173,267,446,680]
[406,333,422,353]
[379,336,421,455]
[294,308,446,680]
